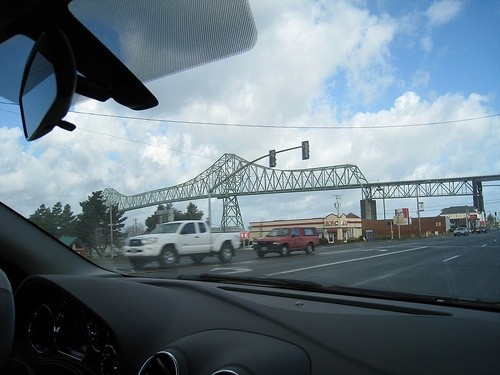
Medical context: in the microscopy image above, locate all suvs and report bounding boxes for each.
[252,226,319,258]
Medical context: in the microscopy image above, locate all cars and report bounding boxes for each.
[453,227,469,237]
[471,226,497,233]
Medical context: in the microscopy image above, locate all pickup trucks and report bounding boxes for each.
[121,219,241,270]
